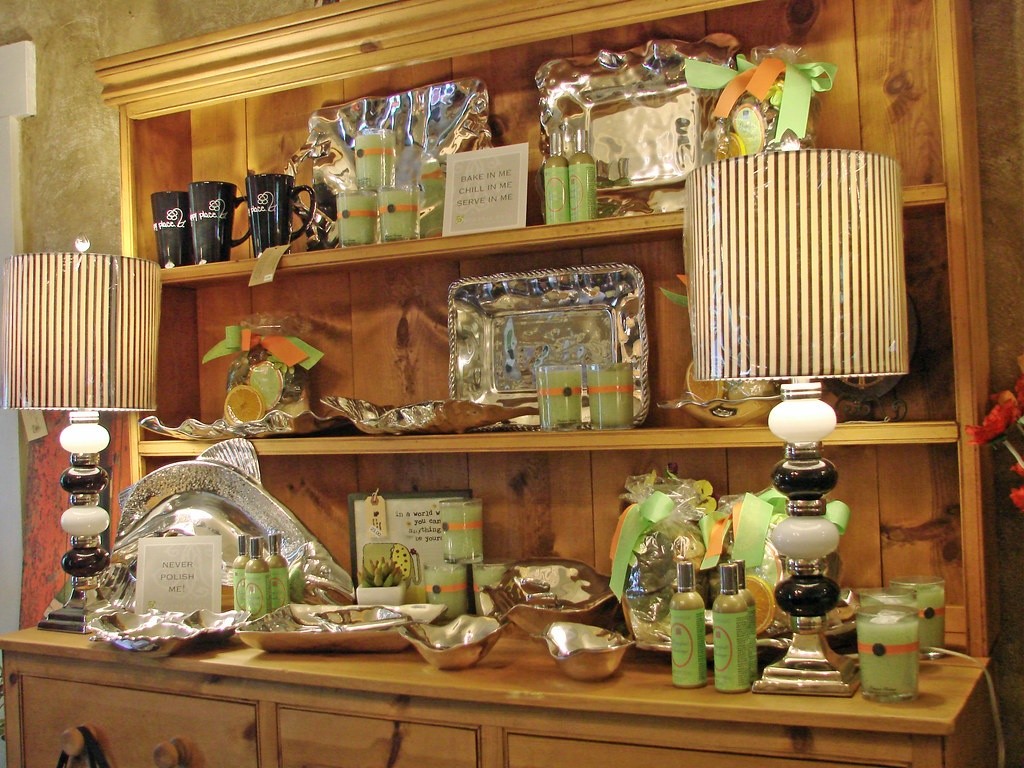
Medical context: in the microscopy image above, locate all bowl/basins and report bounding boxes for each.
[398,555,634,677]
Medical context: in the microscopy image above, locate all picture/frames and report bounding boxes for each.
[348,489,476,616]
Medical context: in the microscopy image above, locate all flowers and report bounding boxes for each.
[964,354,1024,514]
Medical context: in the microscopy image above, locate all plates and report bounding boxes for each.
[656,396,781,422]
[89,606,449,652]
[632,619,857,660]
[448,263,652,431]
[140,394,538,440]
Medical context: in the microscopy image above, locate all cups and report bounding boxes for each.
[441,498,483,564]
[857,587,913,606]
[425,561,468,625]
[536,361,633,431]
[473,558,518,616]
[853,605,921,703]
[889,575,945,658]
[152,128,419,270]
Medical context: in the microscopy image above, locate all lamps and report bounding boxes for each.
[683,128,910,698]
[0,232,162,633]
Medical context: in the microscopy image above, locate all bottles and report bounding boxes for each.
[730,560,759,685]
[234,534,290,620]
[544,128,596,224]
[670,560,706,688]
[715,564,750,691]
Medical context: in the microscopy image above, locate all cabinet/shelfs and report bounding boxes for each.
[0,0,999,767]
